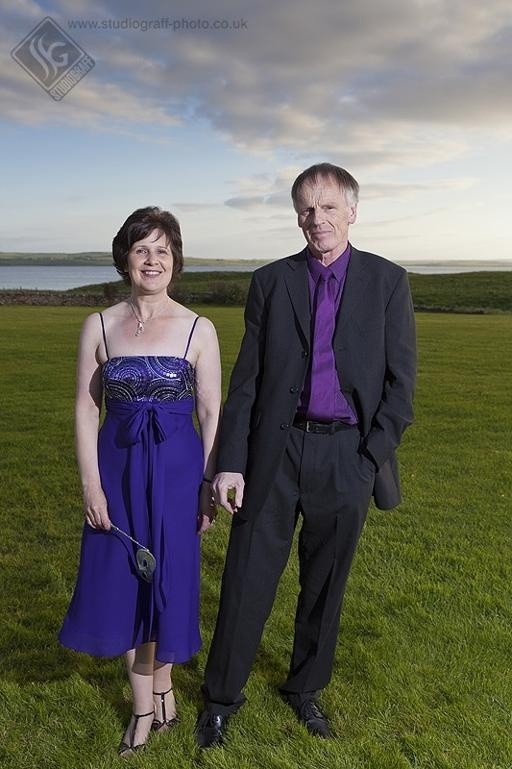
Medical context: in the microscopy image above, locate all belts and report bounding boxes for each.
[292,420,354,436]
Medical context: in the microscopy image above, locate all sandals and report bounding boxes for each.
[152,687,177,734]
[120,702,157,757]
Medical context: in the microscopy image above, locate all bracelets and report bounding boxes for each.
[202,474,211,484]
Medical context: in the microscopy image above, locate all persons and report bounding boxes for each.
[193,163,417,761]
[59,204,221,757]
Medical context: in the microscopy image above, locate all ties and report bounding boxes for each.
[306,269,336,423]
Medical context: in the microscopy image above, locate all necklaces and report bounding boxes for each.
[128,299,169,336]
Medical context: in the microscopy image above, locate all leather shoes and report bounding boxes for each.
[196,709,226,749]
[286,692,330,740]
